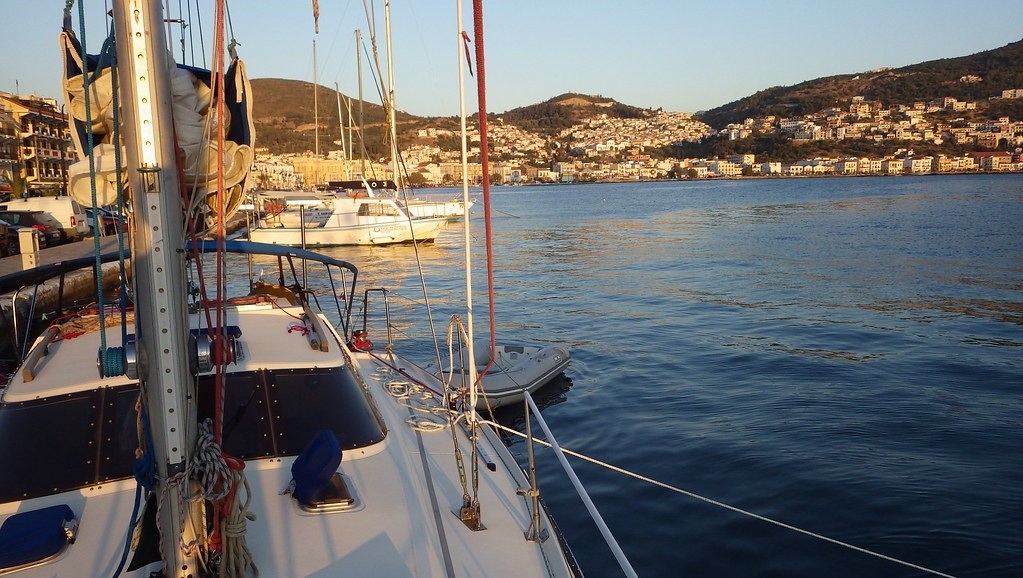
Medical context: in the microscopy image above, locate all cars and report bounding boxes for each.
[0,219,47,257]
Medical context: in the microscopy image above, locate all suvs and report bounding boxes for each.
[0,209,68,247]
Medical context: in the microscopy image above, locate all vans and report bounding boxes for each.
[0,194,88,244]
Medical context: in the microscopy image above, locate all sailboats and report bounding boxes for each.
[0,0,642,578]
